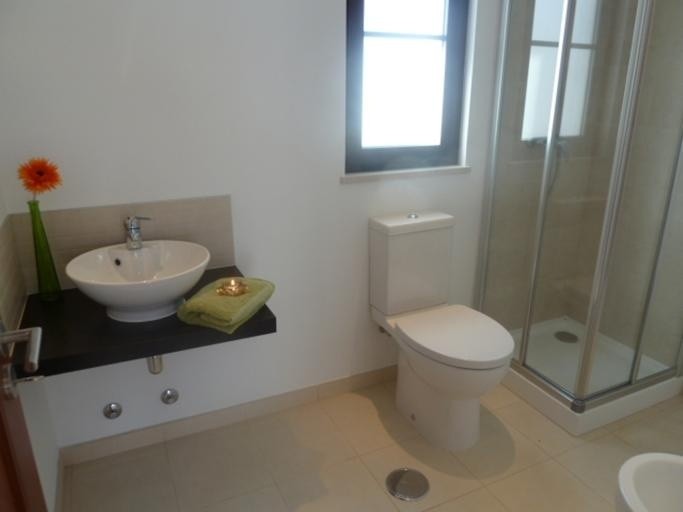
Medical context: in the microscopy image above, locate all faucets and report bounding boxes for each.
[123,215,152,250]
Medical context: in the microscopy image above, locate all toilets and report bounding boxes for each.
[615,451,683,512]
[368,211,516,454]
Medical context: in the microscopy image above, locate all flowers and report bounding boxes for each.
[15,153,64,200]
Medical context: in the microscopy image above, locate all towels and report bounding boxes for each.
[177,274,276,337]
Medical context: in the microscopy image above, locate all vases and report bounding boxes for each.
[24,199,62,302]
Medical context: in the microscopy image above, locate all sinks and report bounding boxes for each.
[65,239,210,323]
